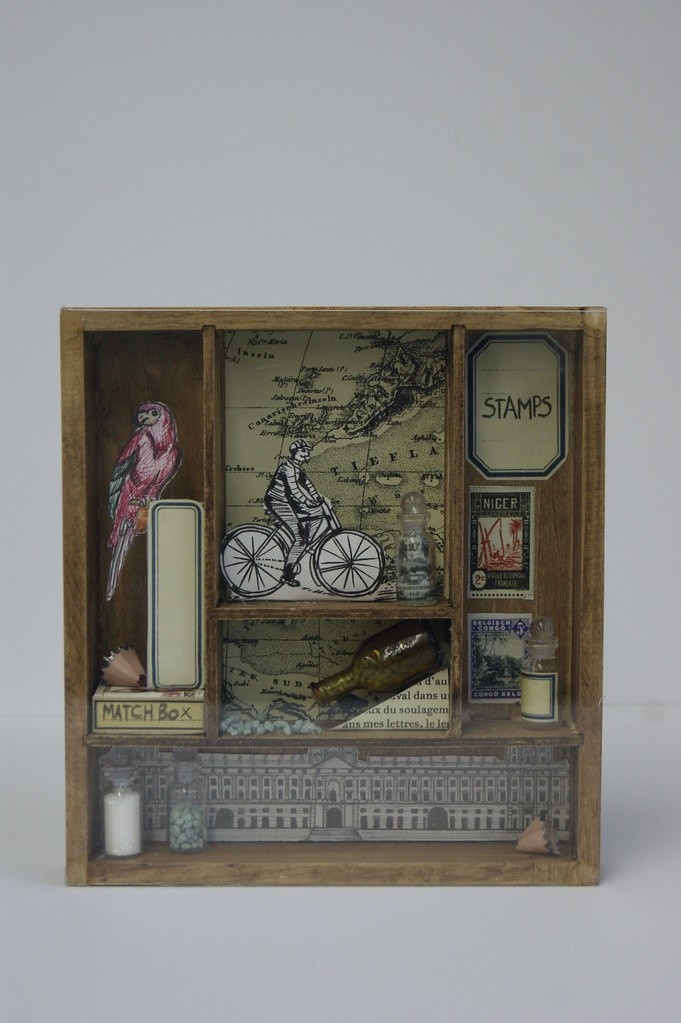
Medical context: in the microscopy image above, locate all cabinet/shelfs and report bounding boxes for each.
[61,306,607,890]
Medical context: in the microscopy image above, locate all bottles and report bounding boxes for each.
[311,622,445,709]
[396,491,437,601]
[166,751,207,853]
[520,617,561,729]
[101,744,142,858]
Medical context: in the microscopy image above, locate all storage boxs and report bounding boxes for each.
[92,678,205,735]
